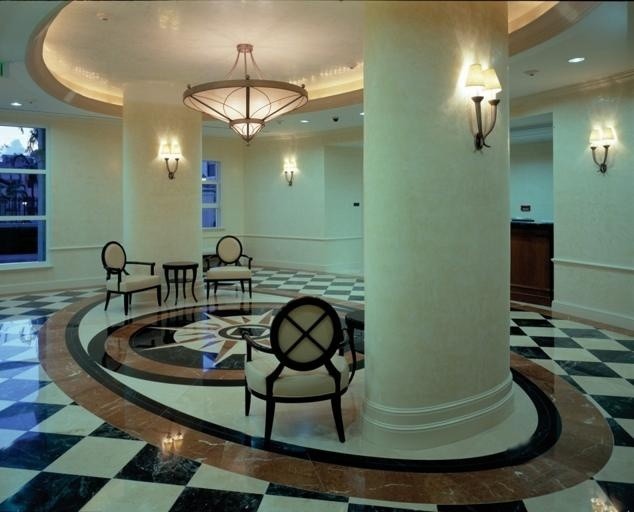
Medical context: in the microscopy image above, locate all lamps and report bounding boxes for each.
[159,144,181,180]
[183,44,308,145]
[589,126,615,175]
[283,162,296,186]
[465,62,503,151]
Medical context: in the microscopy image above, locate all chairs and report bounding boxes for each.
[244,296,357,444]
[101,240,162,316]
[203,235,253,300]
